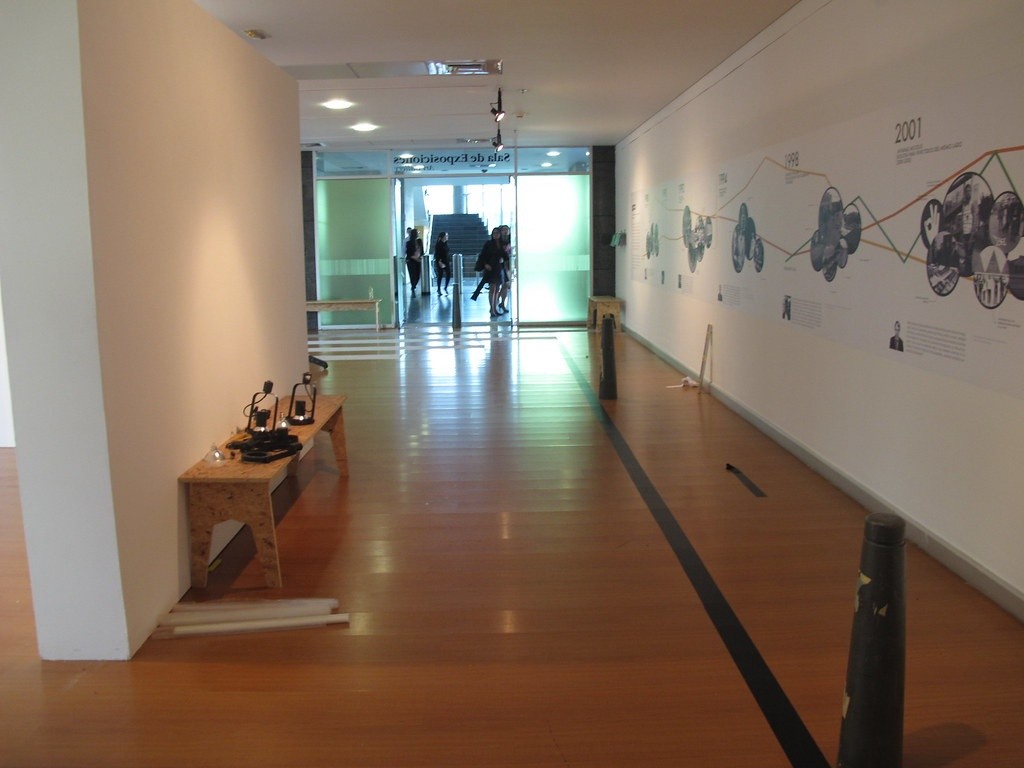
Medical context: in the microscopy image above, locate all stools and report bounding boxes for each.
[587,296,623,332]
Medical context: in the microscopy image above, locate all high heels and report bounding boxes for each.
[489,309,497,316]
[497,312,503,315]
[498,302,509,313]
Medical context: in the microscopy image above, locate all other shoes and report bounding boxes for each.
[412,289,417,298]
[437,290,443,295]
[443,287,448,294]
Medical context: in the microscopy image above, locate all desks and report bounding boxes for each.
[179,394,349,592]
[306,299,381,334]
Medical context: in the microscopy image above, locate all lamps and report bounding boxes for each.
[490,125,505,152]
[490,88,508,124]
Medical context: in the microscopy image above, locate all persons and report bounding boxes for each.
[405,227,412,242]
[889,321,904,352]
[718,285,722,300]
[434,232,451,295]
[474,225,511,317]
[406,229,425,299]
